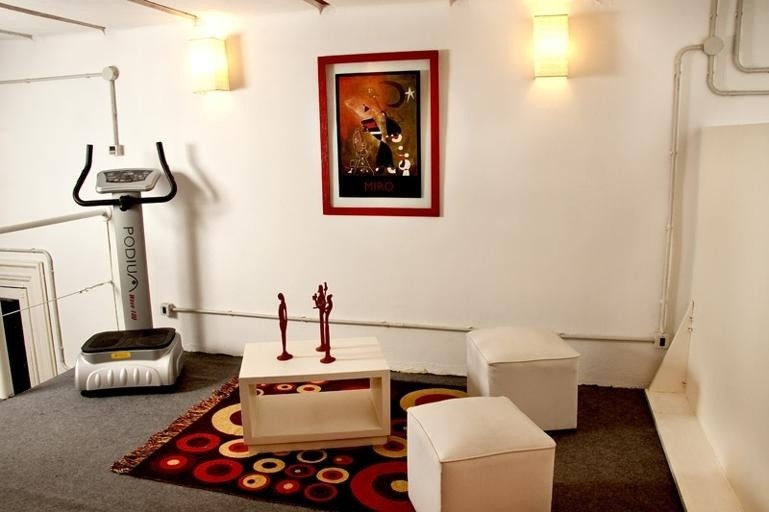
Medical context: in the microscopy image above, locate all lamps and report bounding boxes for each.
[186,37,230,92]
[532,14,569,79]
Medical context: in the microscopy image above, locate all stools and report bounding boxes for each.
[407,396,556,512]
[466,325,581,432]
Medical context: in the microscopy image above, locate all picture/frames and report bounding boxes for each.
[317,49,439,216]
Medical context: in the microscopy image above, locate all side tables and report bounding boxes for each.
[238,336,390,451]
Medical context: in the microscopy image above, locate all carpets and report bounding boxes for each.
[110,376,467,512]
